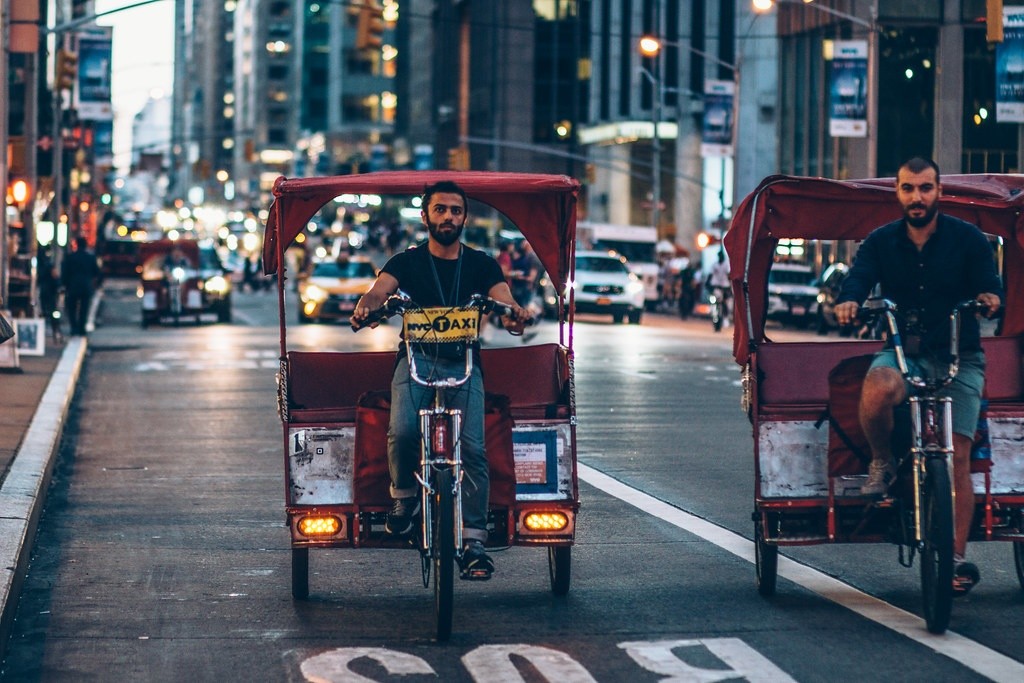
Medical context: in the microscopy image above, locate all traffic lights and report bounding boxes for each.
[357,0,385,48]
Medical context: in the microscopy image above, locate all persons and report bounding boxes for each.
[41,265,65,344]
[8,240,37,317]
[834,157,1004,598]
[671,252,732,328]
[350,182,530,581]
[478,240,545,345]
[61,237,102,335]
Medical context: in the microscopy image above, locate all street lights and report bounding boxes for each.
[753,0,877,179]
[640,37,741,210]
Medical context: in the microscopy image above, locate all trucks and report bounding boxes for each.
[576,222,660,314]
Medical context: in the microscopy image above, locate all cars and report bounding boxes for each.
[768,263,818,326]
[296,256,378,325]
[542,249,647,324]
[140,241,231,329]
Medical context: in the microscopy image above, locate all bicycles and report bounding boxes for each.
[708,288,728,332]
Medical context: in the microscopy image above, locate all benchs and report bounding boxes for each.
[283,345,572,422]
[754,336,1024,415]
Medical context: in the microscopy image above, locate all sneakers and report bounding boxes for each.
[861,458,897,493]
[461,542,495,580]
[385,498,420,534]
[953,556,971,593]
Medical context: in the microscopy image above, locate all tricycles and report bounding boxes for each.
[722,170,1024,636]
[264,172,581,647]
